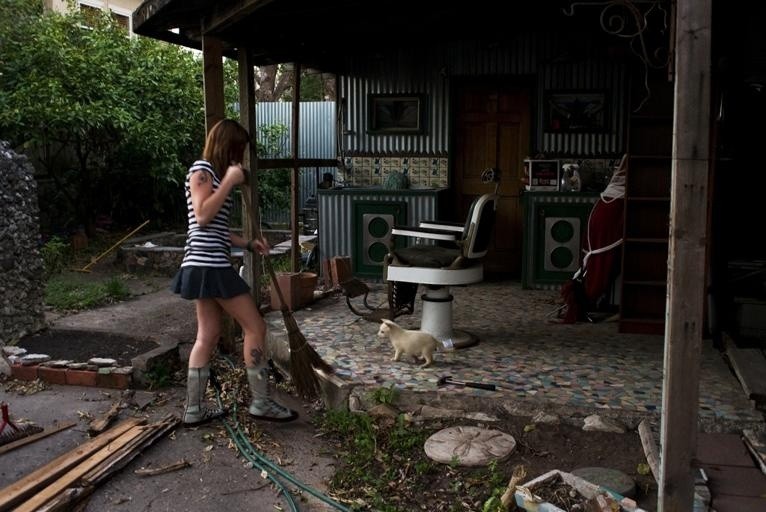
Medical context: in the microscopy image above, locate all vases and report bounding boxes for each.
[271,272,317,310]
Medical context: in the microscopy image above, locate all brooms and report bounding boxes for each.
[233,165,334,399]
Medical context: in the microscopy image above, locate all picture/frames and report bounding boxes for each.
[542,88,610,134]
[367,93,425,136]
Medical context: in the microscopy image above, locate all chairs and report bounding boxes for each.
[340,194,499,348]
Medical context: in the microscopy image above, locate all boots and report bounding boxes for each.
[181,361,228,429]
[247,365,299,423]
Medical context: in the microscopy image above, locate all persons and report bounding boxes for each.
[319,172,334,186]
[169,118,300,428]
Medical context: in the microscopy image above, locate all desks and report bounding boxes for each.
[519,191,600,292]
[318,187,447,284]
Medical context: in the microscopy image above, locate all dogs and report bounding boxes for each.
[377,318,456,370]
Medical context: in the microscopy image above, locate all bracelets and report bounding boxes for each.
[246,240,254,253]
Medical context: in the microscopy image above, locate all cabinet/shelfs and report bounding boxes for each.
[618,59,708,338]
